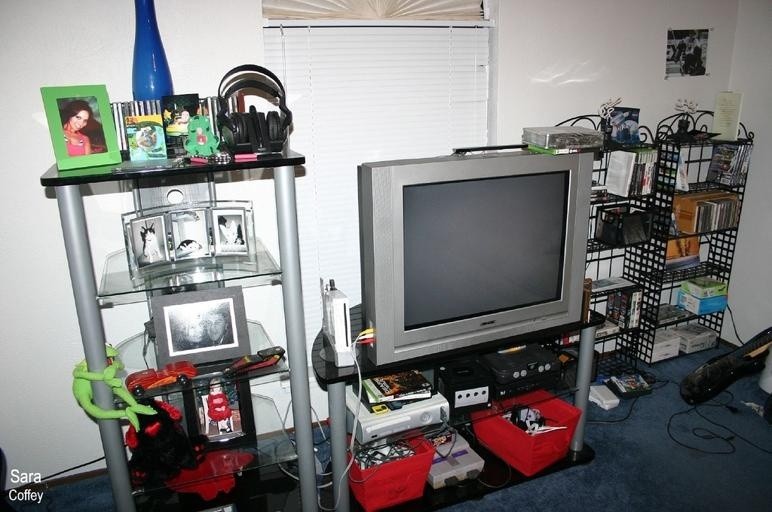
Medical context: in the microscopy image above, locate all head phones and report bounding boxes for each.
[216,63,293,153]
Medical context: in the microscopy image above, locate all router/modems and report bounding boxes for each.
[317,278,355,368]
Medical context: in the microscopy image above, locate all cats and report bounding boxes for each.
[220,220,242,244]
[140,223,164,263]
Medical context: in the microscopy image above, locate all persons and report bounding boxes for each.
[208,378,233,433]
[200,309,234,349]
[59,100,107,157]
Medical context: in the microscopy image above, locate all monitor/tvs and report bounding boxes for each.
[357,152,592,366]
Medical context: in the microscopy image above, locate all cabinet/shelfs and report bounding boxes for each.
[311,303,607,512]
[40,145,318,512]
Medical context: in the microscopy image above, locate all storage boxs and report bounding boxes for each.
[470,388,584,478]
[327,416,436,512]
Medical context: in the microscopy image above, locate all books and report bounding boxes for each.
[706,144,753,187]
[604,147,658,198]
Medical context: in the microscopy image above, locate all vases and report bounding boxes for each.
[132,0,174,102]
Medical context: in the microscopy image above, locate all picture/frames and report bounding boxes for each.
[150,284,252,370]
[180,364,258,453]
[120,199,260,282]
[40,84,123,172]
